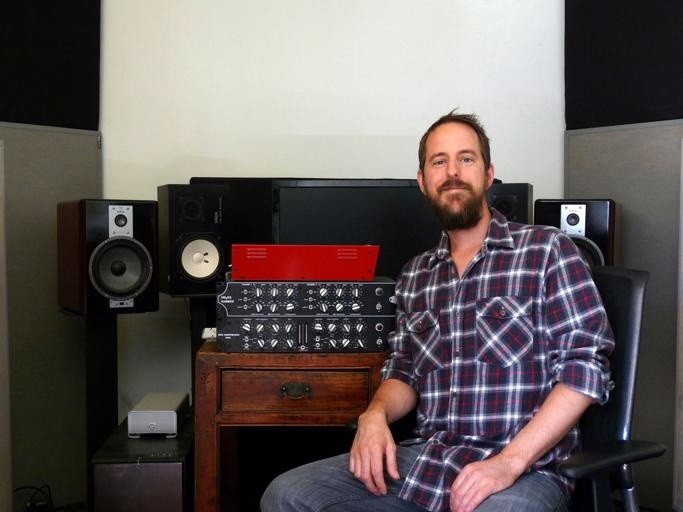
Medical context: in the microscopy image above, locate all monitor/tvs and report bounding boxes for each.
[270,178,497,289]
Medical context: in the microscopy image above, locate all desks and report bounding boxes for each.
[191,338,392,511]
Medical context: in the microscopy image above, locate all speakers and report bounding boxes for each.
[189,177,272,265]
[534,198,616,272]
[90,406,194,512]
[489,183,533,224]
[156,184,230,296]
[56,199,161,316]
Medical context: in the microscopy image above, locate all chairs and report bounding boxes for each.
[553,264,667,511]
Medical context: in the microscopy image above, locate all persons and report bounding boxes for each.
[255,109,617,512]
